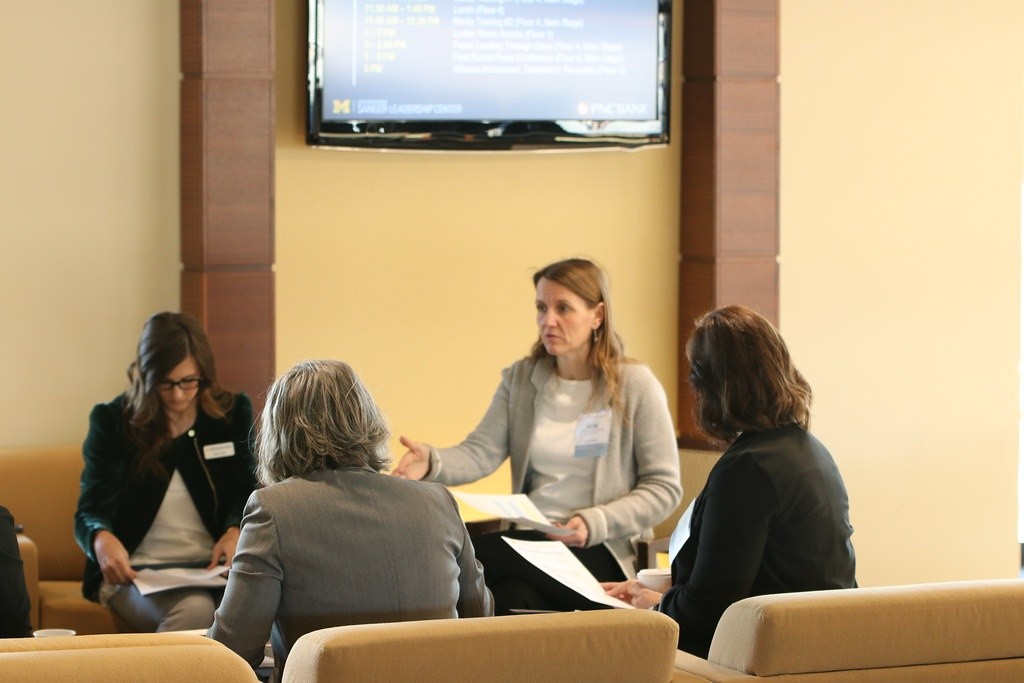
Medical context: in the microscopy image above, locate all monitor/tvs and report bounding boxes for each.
[305,0,672,155]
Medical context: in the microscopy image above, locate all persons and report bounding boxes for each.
[596,305,858,660]
[0,506,34,638]
[390,259,683,616]
[73,313,259,633]
[205,359,494,683]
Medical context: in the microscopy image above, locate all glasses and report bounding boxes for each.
[153,377,203,392]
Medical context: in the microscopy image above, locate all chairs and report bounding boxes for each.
[0,523,1024,683]
